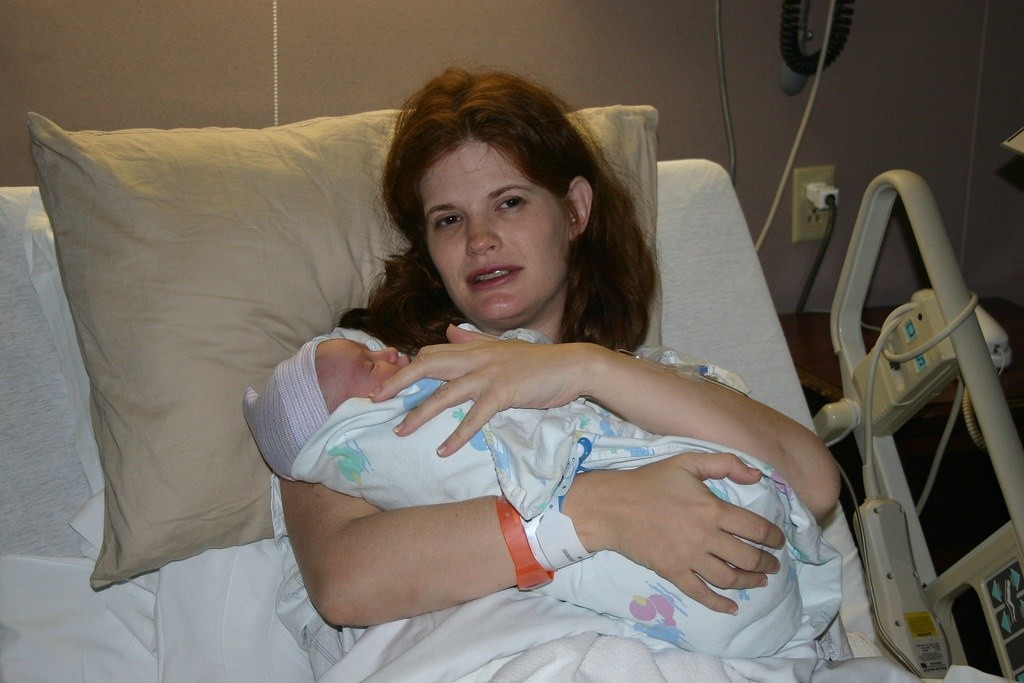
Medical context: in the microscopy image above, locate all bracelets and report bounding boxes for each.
[496,493,552,592]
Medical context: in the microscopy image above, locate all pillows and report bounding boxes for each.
[32,100,659,592]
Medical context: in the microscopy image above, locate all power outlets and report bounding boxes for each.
[792,162,841,243]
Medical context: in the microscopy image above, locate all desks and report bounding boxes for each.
[777,295,1024,526]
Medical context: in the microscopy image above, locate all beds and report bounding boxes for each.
[0,159,1024,683]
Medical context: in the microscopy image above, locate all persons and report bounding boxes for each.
[245,67,841,682]
